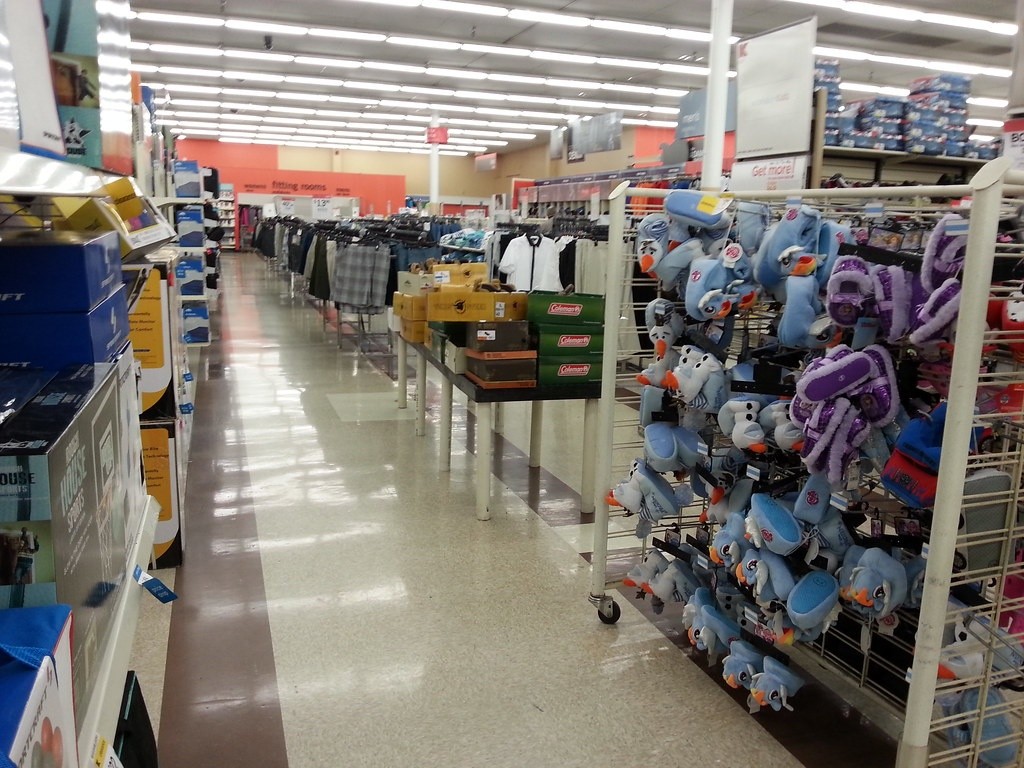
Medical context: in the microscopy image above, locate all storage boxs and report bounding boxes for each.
[813,57,997,159]
[0,176,185,768]
[393,263,603,388]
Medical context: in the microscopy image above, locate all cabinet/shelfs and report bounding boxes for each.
[149,171,240,436]
[0,153,162,768]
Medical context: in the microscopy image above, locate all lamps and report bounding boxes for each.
[814,48,1013,80]
[97,0,741,156]
[793,0,1019,39]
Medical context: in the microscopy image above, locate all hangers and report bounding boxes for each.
[262,216,461,246]
[495,213,608,241]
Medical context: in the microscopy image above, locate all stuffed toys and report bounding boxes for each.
[604,190,1024,762]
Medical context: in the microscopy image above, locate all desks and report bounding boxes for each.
[394,330,603,522]
